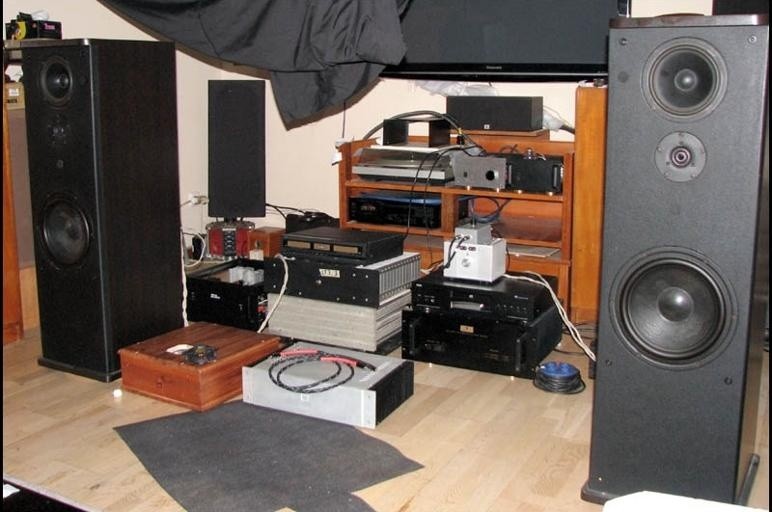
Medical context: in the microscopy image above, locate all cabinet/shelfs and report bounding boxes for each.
[118,318,278,415]
[333,126,574,324]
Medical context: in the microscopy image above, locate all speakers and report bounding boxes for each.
[21,38,181,386]
[440,98,542,133]
[206,77,268,217]
[583,16,768,509]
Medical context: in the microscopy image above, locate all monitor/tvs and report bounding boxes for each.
[384,2,629,85]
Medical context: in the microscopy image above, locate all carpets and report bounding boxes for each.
[112,399,424,512]
